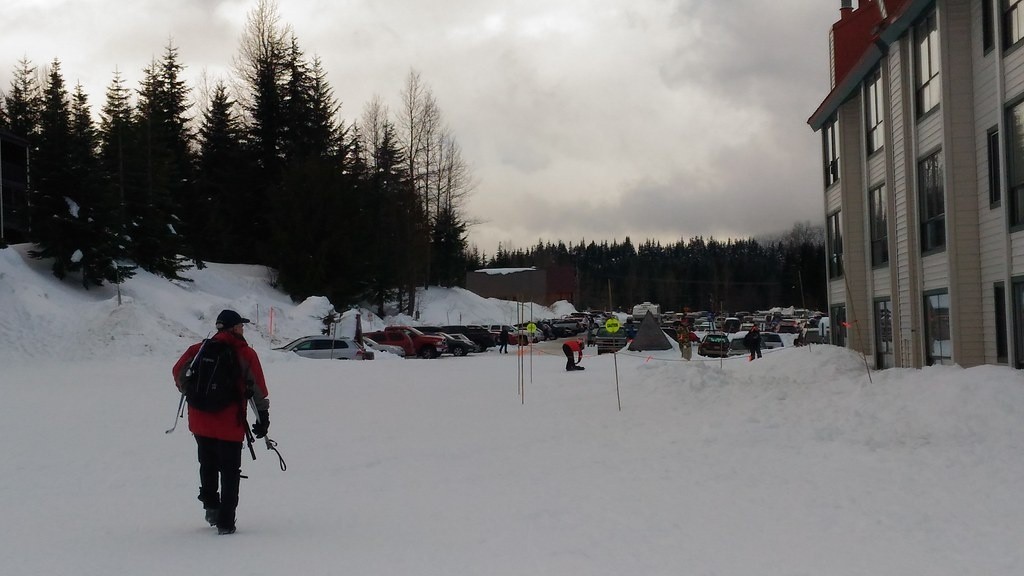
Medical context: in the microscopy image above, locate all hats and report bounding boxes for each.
[216,310,249,331]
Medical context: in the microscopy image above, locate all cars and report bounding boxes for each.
[268,307,831,361]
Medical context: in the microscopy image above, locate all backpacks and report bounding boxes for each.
[186,339,242,412]
[743,332,751,354]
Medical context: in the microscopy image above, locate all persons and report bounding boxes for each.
[562,340,585,371]
[749,324,761,362]
[172,310,270,534]
[498,326,509,354]
[796,330,803,347]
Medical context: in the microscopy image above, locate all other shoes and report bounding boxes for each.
[204,507,236,534]
[505,352,508,353]
[499,349,501,353]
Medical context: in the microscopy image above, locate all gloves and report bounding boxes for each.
[251,410,270,438]
